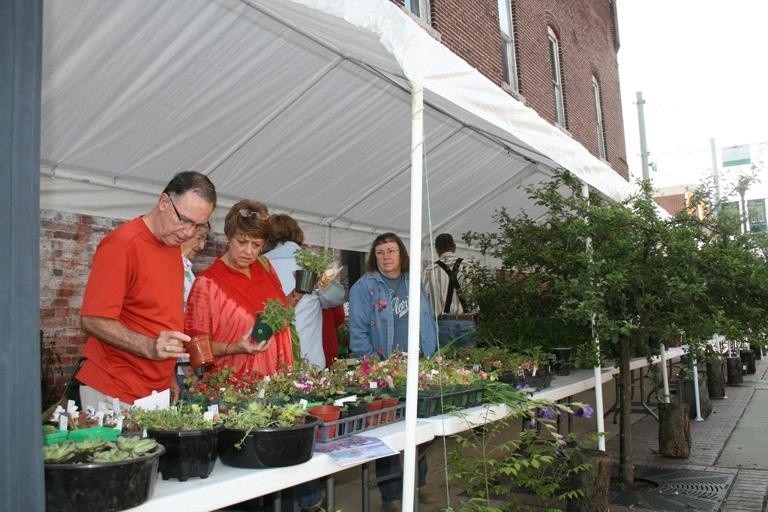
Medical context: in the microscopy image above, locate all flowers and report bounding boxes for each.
[179,335,593,456]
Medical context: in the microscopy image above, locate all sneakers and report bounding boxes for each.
[379,495,402,512]
[417,483,435,505]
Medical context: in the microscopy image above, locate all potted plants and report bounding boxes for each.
[294,248,332,292]
[44,437,166,512]
[217,401,320,468]
[130,404,225,481]
[252,299,295,342]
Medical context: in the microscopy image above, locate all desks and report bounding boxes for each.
[119,420,434,512]
[601,335,726,424]
[418,368,612,465]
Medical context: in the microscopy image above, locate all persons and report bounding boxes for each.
[183,198,334,511]
[74,169,195,431]
[349,231,444,510]
[174,219,213,402]
[423,232,489,438]
[321,303,346,372]
[261,209,346,377]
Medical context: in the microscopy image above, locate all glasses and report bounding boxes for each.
[165,192,211,233]
[192,235,208,245]
[238,208,270,221]
[374,249,400,258]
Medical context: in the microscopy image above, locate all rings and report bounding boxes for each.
[164,344,171,352]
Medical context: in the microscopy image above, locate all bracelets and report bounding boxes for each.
[292,289,301,303]
[222,341,231,355]
